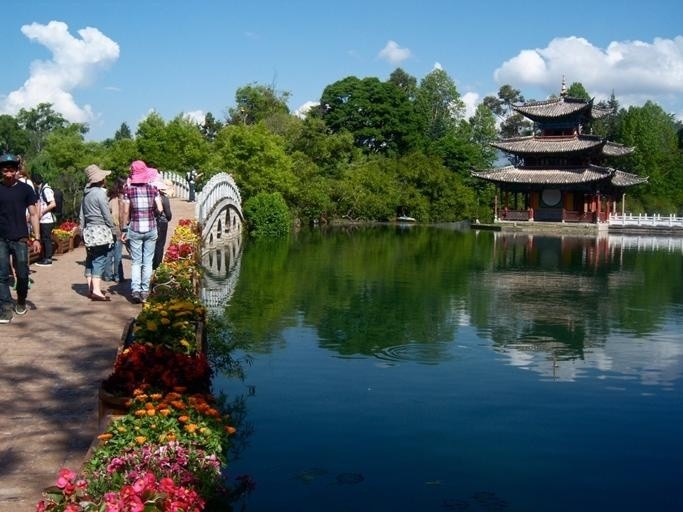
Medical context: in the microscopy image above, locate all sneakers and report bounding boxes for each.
[87,290,110,301]
[0,302,28,324]
[36,258,53,264]
[131,291,150,304]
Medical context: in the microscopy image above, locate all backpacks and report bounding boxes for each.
[42,187,63,214]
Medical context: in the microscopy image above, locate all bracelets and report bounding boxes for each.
[34,239,40,242]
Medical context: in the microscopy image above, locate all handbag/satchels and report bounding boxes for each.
[82,224,114,253]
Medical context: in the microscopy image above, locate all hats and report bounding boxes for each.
[0,153,19,163]
[84,164,112,188]
[130,160,175,197]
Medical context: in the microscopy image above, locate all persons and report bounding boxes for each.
[187,167,204,203]
[30,172,57,266]
[77,160,171,305]
[0,153,42,324]
[8,154,34,291]
